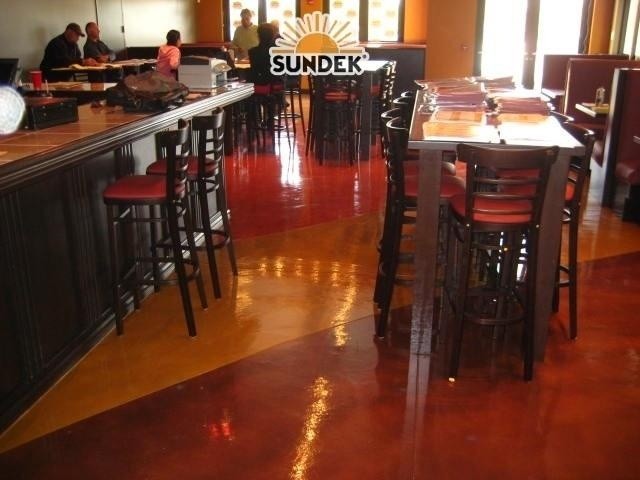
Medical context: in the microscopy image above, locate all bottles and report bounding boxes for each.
[595,88,606,108]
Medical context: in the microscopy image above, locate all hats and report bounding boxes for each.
[66,23,85,37]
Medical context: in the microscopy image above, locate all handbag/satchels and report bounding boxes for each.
[106,71,190,112]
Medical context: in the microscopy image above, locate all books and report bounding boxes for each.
[413,71,576,150]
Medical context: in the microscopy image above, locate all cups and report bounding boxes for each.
[30,70,42,90]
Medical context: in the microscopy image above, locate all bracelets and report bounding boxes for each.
[108,56,110,60]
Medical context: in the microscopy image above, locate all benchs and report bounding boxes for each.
[544,46,640,221]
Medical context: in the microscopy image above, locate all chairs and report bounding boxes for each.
[105,116,209,345]
[145,107,250,301]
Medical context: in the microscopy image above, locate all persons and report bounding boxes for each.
[156,30,182,82]
[41,22,98,81]
[84,22,117,81]
[232,9,289,129]
